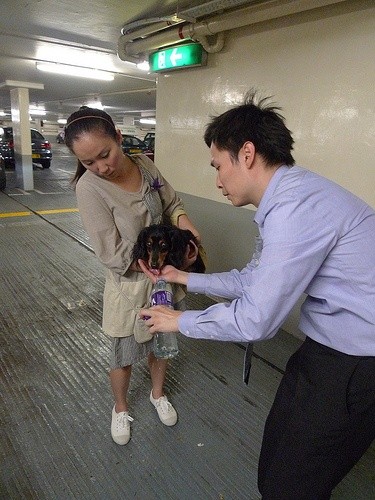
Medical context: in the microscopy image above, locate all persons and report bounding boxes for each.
[138,86,375,500]
[64,103,200,448]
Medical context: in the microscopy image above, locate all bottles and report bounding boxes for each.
[151,277,179,360]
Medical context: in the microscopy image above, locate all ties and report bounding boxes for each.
[243,343,253,386]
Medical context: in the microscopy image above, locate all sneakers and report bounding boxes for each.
[150,388,178,427]
[111,403,134,446]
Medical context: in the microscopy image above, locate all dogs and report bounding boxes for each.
[131,224,206,273]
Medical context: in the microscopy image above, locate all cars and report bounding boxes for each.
[143,133,155,152]
[121,134,154,162]
[0,126,52,169]
[56,130,66,144]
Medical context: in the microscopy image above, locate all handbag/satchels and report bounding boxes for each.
[133,313,153,343]
